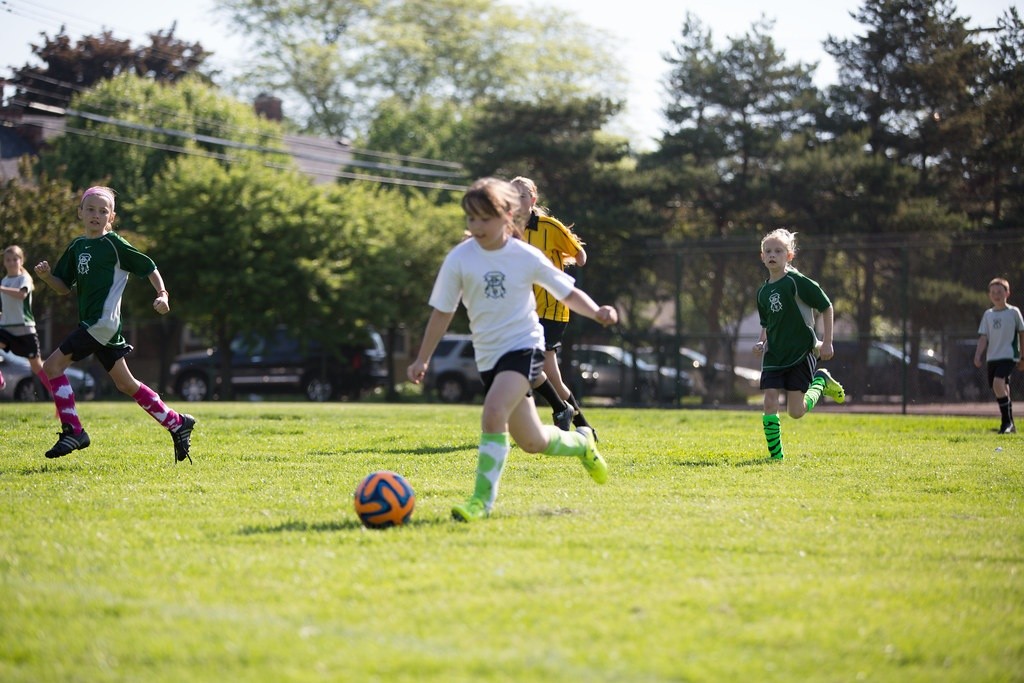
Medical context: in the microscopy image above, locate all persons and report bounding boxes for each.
[0,245,52,396]
[755,229,846,462]
[974,277,1024,434]
[34,186,195,462]
[408,176,608,523]
[512,177,599,443]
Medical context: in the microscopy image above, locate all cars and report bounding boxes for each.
[549,345,694,407]
[0,350,96,402]
[643,347,764,405]
[953,339,1024,402]
[814,341,944,404]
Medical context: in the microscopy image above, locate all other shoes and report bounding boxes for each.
[997,422,1016,434]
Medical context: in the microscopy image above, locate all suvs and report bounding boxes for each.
[428,331,488,403]
[168,315,389,402]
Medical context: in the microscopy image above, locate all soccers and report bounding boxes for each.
[353,469,417,529]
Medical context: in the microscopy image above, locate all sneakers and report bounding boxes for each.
[590,428,598,442]
[577,426,607,484]
[44,421,90,459]
[170,413,195,465]
[452,497,487,524]
[814,368,846,404]
[551,400,574,430]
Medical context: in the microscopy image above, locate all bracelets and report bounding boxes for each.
[158,290,168,296]
